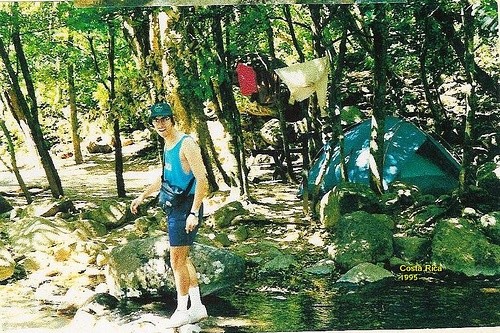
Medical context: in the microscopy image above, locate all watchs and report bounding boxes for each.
[190,212,199,217]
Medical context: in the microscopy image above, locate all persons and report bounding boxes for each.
[130,103,210,329]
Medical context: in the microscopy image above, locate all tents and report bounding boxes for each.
[297,115,463,200]
